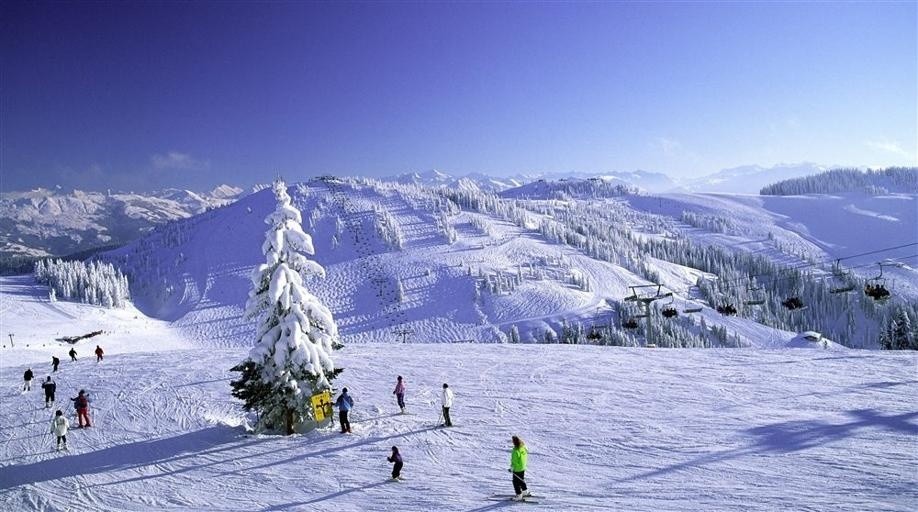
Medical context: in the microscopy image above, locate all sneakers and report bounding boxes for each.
[511,490,531,502]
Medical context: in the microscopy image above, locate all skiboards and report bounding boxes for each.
[483,494,547,505]
[340,428,361,437]
[389,411,418,415]
[383,476,415,484]
[422,423,463,427]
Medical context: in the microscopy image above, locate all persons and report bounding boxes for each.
[871,285,881,300]
[506,435,531,503]
[865,284,878,300]
[68,347,77,362]
[439,382,453,427]
[333,387,354,434]
[785,297,804,310]
[94,345,104,362]
[22,366,34,391]
[724,303,736,316]
[49,410,70,452]
[385,446,406,484]
[879,284,889,297]
[874,284,885,299]
[661,307,678,320]
[391,375,407,415]
[50,355,59,372]
[40,376,56,408]
[70,389,93,428]
